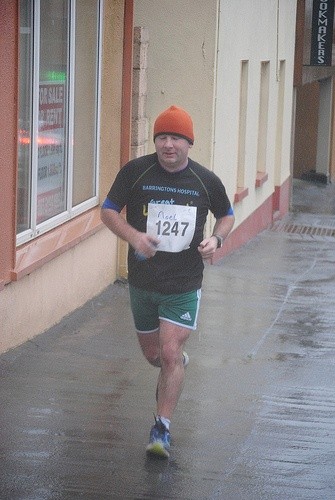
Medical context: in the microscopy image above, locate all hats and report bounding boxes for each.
[154,105,194,144]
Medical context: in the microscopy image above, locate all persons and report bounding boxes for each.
[100,106,234,458]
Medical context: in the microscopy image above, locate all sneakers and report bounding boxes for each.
[145,412,171,458]
[156,350,190,402]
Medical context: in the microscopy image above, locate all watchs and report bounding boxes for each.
[211,233,223,248]
